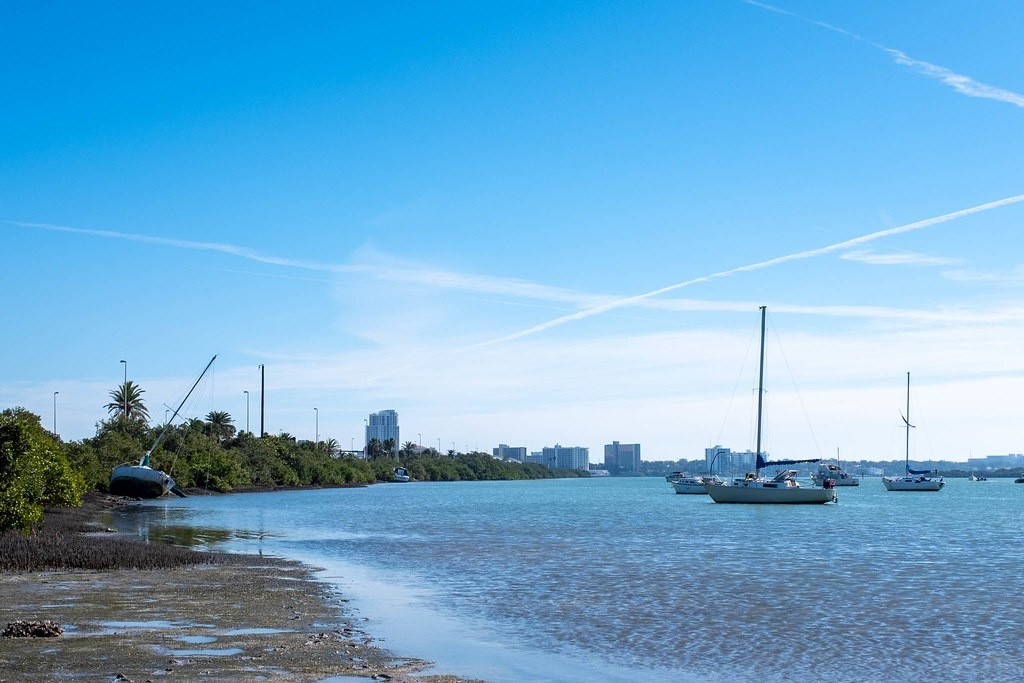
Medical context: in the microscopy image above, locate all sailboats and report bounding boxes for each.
[104,351,218,498]
[701,307,838,504]
[882,369,946,493]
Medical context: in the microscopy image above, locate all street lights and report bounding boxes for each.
[53,391,59,434]
[364,419,368,460]
[418,433,421,456]
[120,360,128,433]
[313,407,319,445]
[438,437,440,455]
[244,390,250,435]
[166,409,169,424]
[352,437,354,451]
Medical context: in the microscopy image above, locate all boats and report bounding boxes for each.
[665,472,685,483]
[671,477,723,496]
[814,448,860,487]
